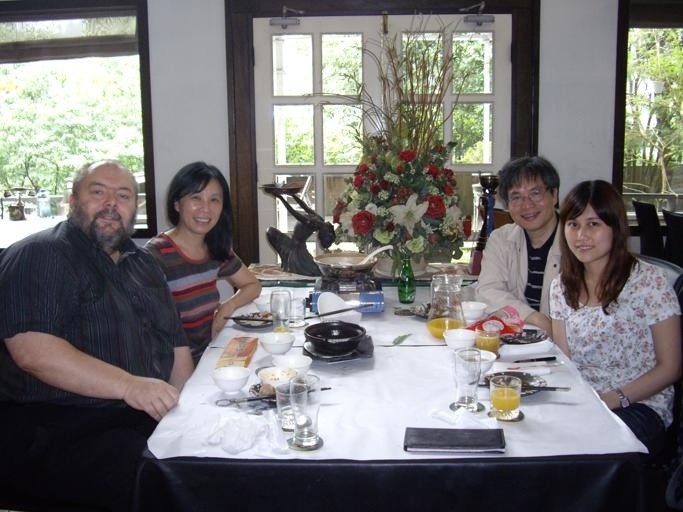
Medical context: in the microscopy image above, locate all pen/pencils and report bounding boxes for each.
[514,357,557,363]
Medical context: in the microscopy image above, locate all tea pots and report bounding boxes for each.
[427,274,465,340]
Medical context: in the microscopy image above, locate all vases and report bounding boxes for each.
[374,253,427,279]
[8,205,25,220]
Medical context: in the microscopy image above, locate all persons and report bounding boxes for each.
[143,161,263,369]
[548,179,682,463]
[266,194,336,277]
[475,155,563,339]
[1,159,194,512]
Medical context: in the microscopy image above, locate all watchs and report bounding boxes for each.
[616,387,630,408]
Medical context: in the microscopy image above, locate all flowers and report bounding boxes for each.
[323,6,473,261]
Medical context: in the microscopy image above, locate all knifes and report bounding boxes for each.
[513,356,557,362]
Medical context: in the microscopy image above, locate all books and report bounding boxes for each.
[403,426,507,452]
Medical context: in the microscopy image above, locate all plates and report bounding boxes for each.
[252,385,309,402]
[300,342,357,359]
[498,329,547,344]
[499,339,553,355]
[234,313,275,329]
[484,372,546,397]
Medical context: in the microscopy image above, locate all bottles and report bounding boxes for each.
[399,255,416,301]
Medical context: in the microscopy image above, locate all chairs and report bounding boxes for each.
[660,207,682,267]
[632,250,683,297]
[632,199,665,257]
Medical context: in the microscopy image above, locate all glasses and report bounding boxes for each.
[508,185,552,205]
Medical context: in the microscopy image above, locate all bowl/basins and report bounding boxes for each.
[441,329,475,349]
[462,302,487,322]
[455,349,497,376]
[313,253,378,280]
[258,333,297,354]
[305,318,366,354]
[253,365,298,388]
[213,366,249,395]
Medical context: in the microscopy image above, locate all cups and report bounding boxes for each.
[476,331,501,352]
[274,384,306,431]
[288,297,306,326]
[489,376,522,421]
[451,349,482,410]
[290,375,322,449]
[270,290,290,333]
